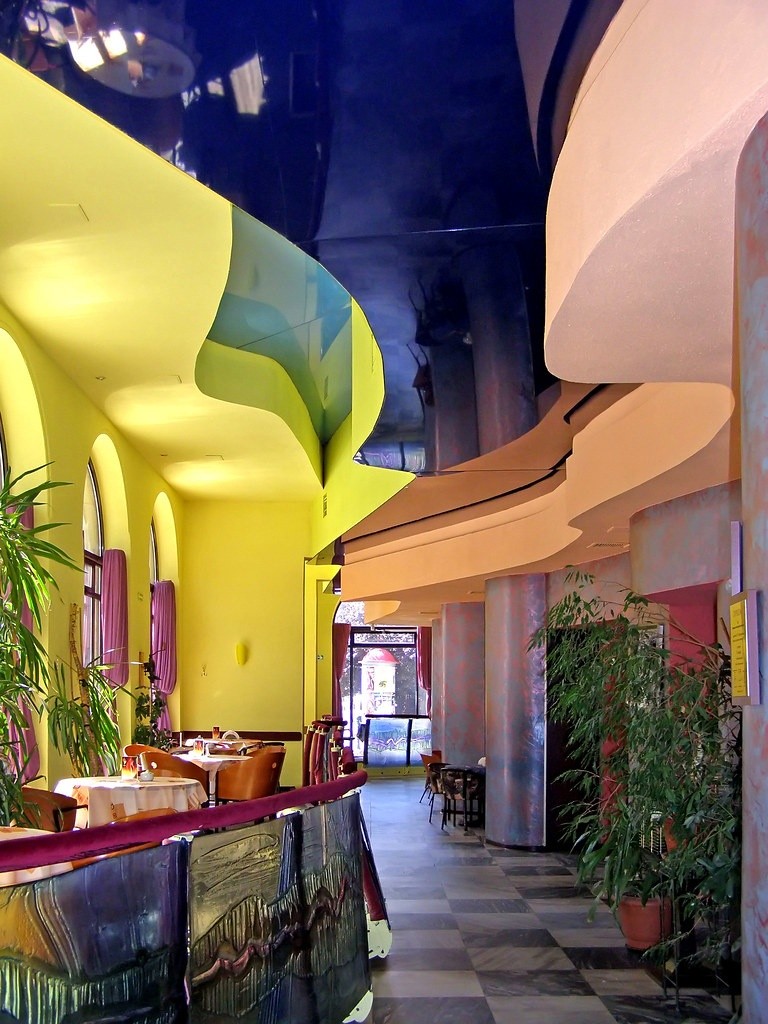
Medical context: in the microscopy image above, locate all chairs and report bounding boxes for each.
[14,742,286,833]
[418,750,485,832]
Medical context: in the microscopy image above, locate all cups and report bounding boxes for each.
[141,772,154,781]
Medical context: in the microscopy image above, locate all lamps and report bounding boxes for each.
[406,276,457,390]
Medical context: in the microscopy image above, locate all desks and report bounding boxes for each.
[53,776,208,831]
[175,755,252,795]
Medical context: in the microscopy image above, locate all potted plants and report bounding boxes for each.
[571,797,671,949]
